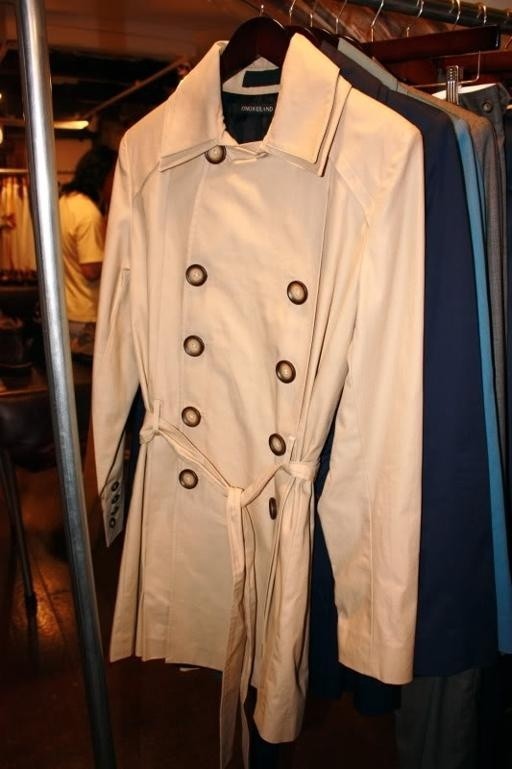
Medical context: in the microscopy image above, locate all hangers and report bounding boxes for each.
[213,2,512,78]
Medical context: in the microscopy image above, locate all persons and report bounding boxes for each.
[51,147,119,462]
